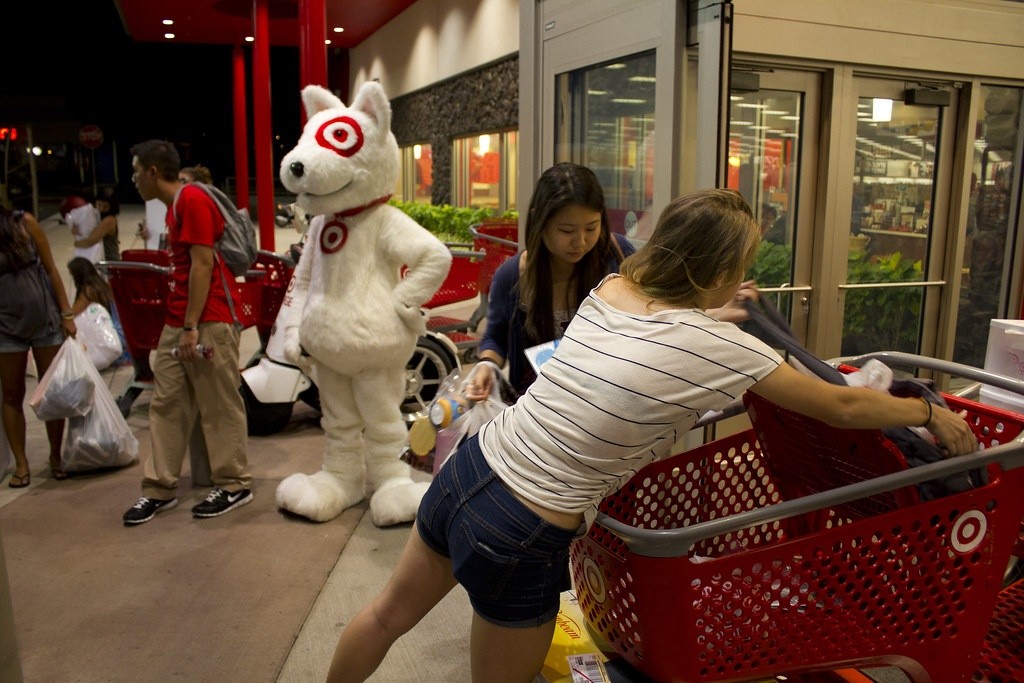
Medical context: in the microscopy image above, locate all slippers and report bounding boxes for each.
[51,469,69,480]
[9,473,30,488]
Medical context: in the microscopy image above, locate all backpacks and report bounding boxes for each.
[173,181,257,276]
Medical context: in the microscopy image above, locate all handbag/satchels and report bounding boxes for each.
[28,335,96,421]
[60,339,138,473]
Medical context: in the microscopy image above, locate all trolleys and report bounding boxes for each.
[568,350,1023,683]
[91,220,522,439]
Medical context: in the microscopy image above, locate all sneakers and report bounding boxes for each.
[191,487,254,516]
[123,495,178,523]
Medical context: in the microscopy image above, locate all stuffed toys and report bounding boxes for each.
[276,81,453,527]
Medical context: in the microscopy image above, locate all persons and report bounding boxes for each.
[0,205,77,488]
[466,162,638,406]
[67,257,131,367]
[71,193,121,262]
[326,188,979,683]
[123,141,254,524]
[137,165,212,238]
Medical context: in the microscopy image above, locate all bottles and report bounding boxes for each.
[170,345,214,359]
[429,395,469,427]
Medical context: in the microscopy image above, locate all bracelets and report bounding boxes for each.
[915,397,932,428]
[183,327,197,330]
[61,311,75,319]
